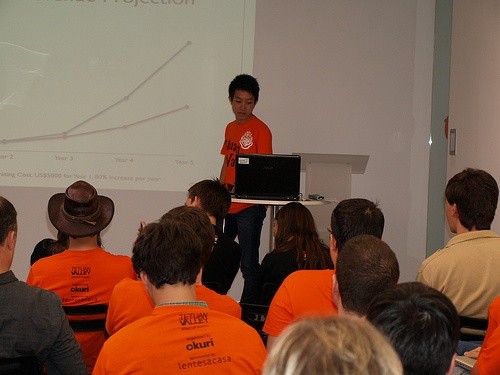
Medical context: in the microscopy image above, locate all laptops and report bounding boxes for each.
[234,153,301,201]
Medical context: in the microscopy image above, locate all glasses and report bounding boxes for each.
[327,225,338,248]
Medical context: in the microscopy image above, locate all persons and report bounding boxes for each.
[25,167,500,375]
[219,73,273,281]
[0,196,88,375]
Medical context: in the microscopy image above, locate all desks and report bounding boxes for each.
[230,197,332,253]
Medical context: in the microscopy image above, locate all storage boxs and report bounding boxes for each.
[235,154,300,201]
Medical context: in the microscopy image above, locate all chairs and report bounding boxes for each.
[61,303,489,343]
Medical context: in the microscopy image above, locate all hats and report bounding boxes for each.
[48,181,114,236]
